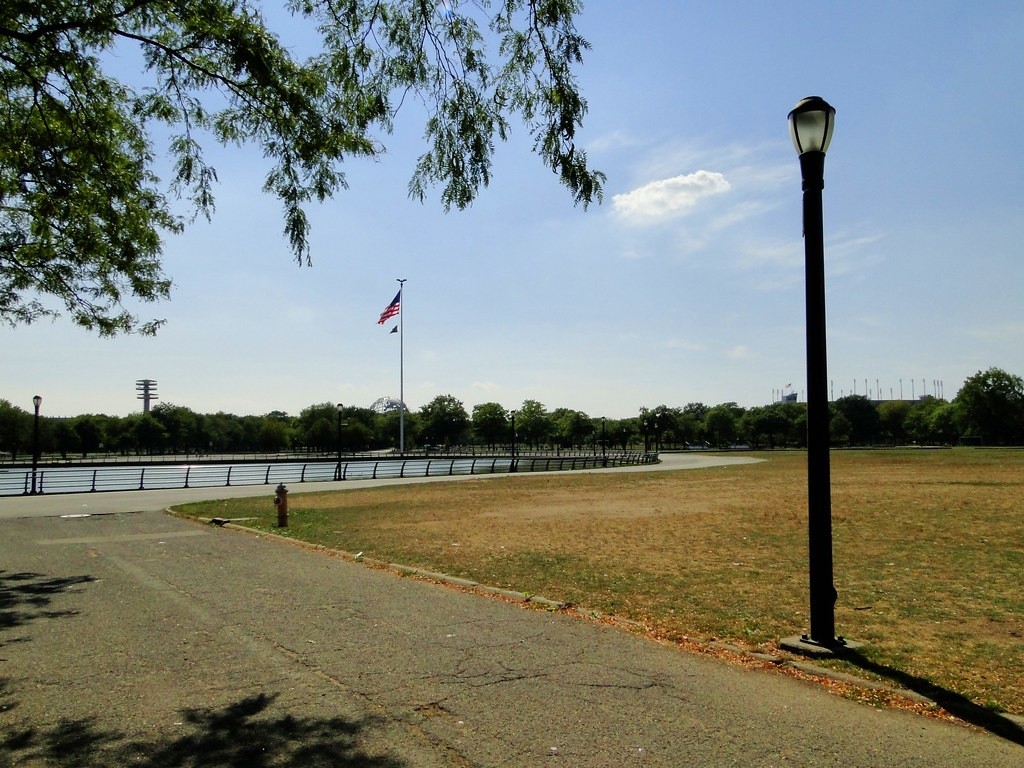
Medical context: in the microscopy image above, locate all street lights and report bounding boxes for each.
[785,91,863,656]
[510,410,515,469]
[30,393,43,496]
[601,416,607,457]
[336,403,344,480]
[592,430,597,457]
[643,420,649,463]
[654,422,660,453]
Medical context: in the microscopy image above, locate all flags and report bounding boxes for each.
[390,326,398,333]
[378,290,400,324]
[785,383,791,388]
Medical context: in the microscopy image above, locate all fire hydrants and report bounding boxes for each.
[273,481,289,527]
